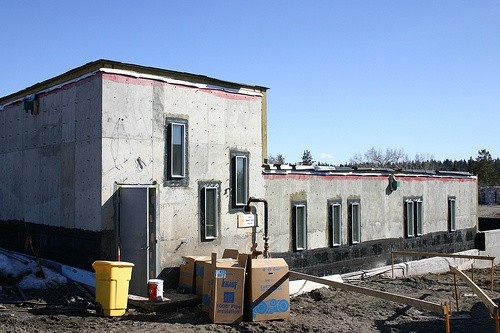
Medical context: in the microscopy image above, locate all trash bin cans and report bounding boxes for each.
[92,260,135,317]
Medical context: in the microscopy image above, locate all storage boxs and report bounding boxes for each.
[179,249,238,312]
[210,251,250,325]
[246,253,291,321]
